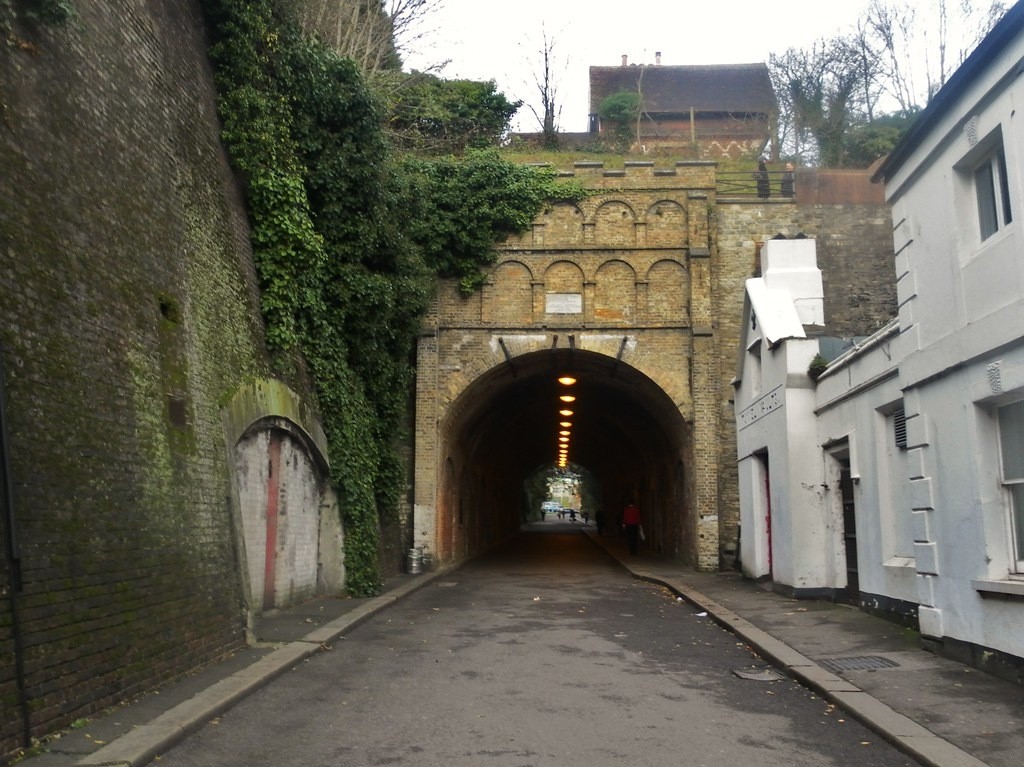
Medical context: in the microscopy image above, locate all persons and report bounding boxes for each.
[781,161,796,197]
[757,155,771,197]
[622,501,642,555]
[541,509,546,521]
[584,513,588,524]
[595,507,606,534]
[557,509,565,519]
[570,510,575,519]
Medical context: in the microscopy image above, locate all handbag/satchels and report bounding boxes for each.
[639,525,646,541]
